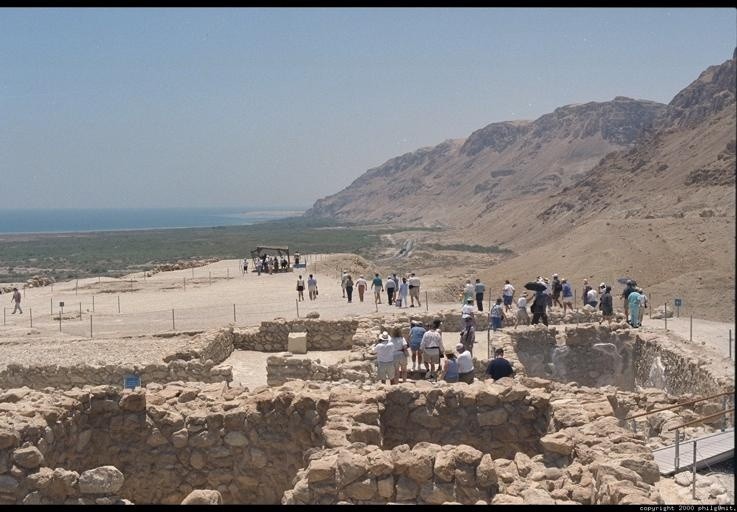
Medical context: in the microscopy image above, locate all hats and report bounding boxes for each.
[378,331,392,342]
[444,349,455,355]
[520,291,528,297]
[598,282,605,287]
[466,296,474,302]
[551,273,558,278]
[455,343,463,350]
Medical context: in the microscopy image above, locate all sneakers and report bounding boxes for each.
[425,371,435,380]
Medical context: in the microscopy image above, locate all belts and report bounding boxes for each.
[424,346,439,349]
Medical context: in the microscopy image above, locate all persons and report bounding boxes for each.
[12,289,23,314]
[244,251,650,386]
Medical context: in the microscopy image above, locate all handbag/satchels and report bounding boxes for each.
[644,304,647,309]
[403,347,411,358]
[395,299,403,307]
[315,290,319,296]
